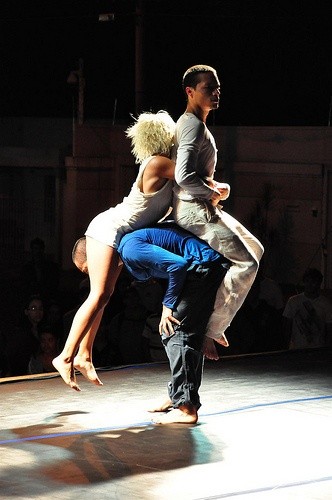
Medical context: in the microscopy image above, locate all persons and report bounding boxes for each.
[92,280,169,367]
[282,266,332,349]
[172,64,264,360]
[0,237,88,375]
[72,223,232,424]
[52,109,230,391]
[216,269,283,356]
